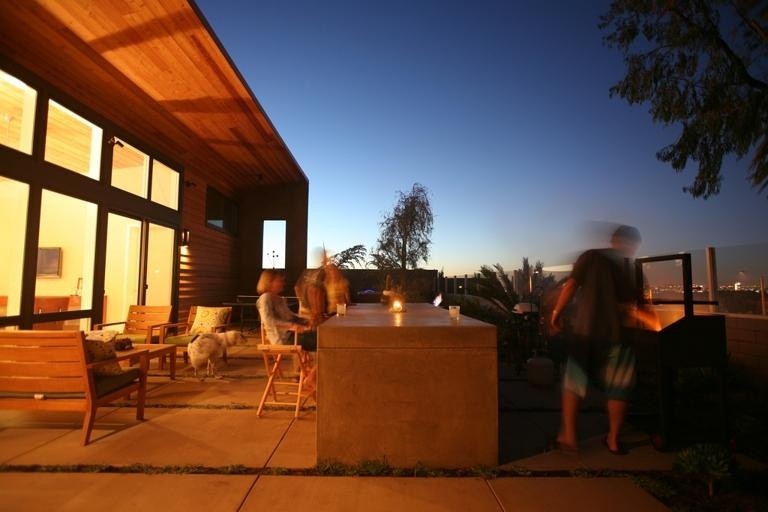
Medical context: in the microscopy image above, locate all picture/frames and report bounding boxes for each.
[36,247,62,278]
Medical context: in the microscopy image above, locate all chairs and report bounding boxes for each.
[93,304,233,370]
[256,317,316,419]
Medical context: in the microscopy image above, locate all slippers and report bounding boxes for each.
[602,435,624,456]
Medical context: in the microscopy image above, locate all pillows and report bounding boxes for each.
[85,335,124,376]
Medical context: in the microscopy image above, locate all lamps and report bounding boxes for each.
[180,229,192,246]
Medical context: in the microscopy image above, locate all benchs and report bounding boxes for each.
[0,329,149,446]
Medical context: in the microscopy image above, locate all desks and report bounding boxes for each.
[221,302,298,333]
[315,302,499,469]
[115,343,177,401]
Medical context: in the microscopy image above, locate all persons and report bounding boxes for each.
[550,225,663,453]
[254,266,317,391]
[323,261,353,317]
[294,267,327,325]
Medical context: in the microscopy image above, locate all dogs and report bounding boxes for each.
[187,330,248,377]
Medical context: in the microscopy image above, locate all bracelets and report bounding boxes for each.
[551,309,560,316]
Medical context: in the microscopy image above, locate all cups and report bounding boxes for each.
[336,303,347,317]
[448,305,461,321]
